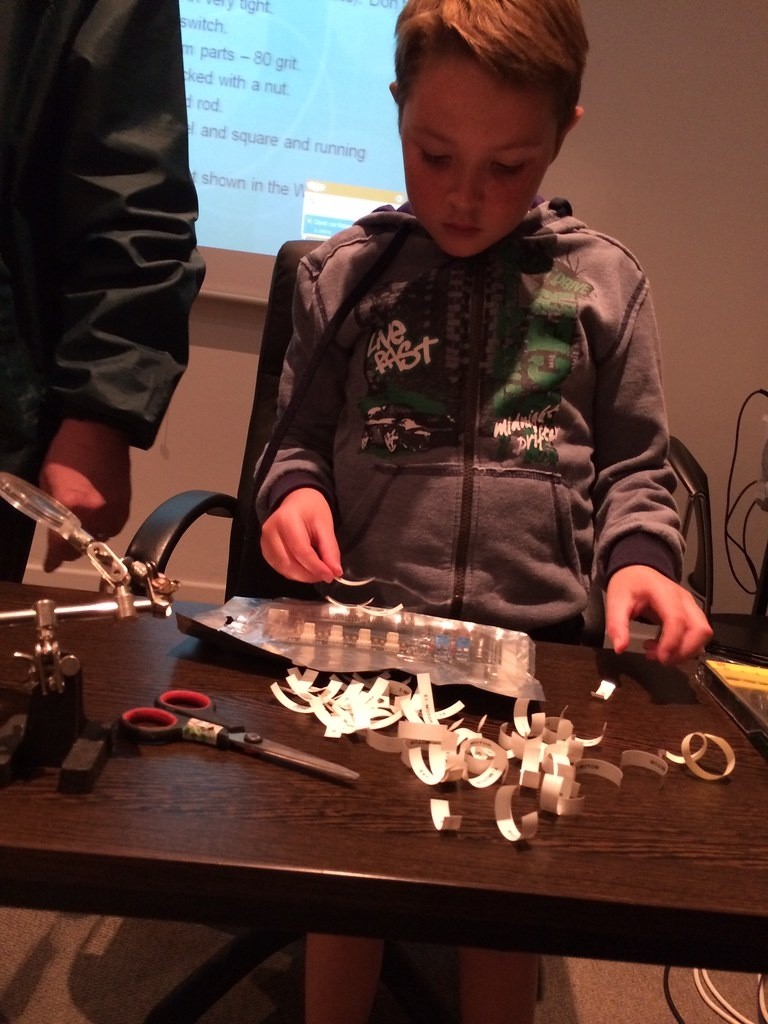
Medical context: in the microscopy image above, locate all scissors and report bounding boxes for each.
[120,688,360,782]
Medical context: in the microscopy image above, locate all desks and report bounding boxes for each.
[1,581,768,975]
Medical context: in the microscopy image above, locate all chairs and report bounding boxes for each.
[122,239,544,1024]
[665,434,767,669]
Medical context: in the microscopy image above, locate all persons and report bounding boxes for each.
[1,0,208,585]
[252,0,714,1024]
